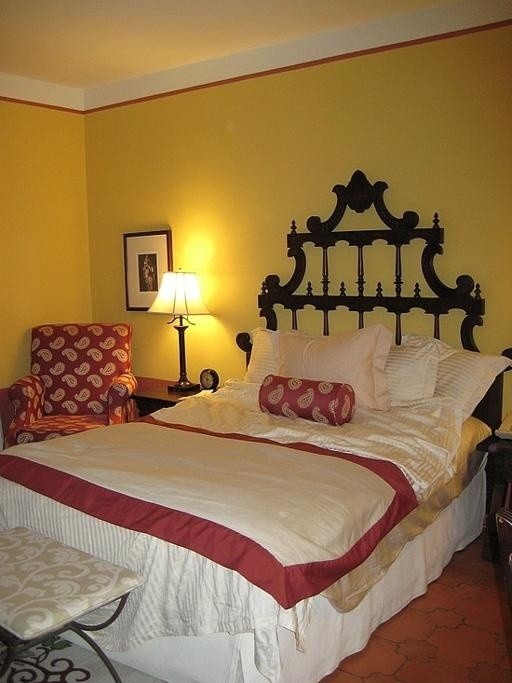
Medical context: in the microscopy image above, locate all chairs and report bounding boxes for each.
[6,322,138,451]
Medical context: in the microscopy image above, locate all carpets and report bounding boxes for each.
[0,627,170,683]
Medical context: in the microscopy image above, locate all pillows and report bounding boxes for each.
[387,343,441,406]
[243,327,277,380]
[274,319,392,411]
[399,334,512,426]
[257,376,356,426]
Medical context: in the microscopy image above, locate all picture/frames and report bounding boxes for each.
[123,229,173,312]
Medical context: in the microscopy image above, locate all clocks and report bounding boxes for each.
[199,368,219,392]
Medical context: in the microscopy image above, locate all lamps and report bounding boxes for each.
[146,272,211,392]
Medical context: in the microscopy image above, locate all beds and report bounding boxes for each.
[0,169,512,683]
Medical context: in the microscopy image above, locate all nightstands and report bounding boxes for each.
[474,433,512,563]
[129,376,220,417]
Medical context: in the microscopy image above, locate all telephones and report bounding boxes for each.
[495,414,512,439]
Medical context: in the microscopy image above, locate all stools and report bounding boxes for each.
[0,527,147,683]
